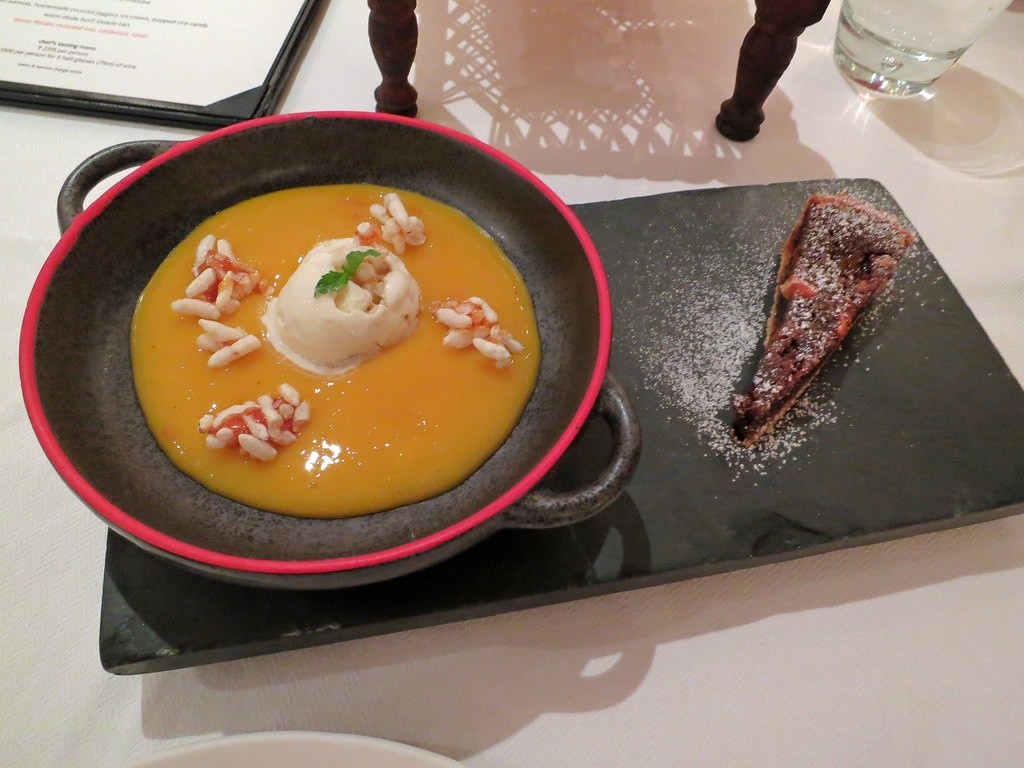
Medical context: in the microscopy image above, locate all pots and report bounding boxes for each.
[18,109,642,591]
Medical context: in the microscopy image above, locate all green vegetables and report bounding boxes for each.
[313,249,381,298]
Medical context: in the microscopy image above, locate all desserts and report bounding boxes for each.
[727,193,912,446]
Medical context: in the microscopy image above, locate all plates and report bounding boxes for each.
[97,177,1023,677]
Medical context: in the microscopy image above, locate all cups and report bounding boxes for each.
[833,0,1012,99]
